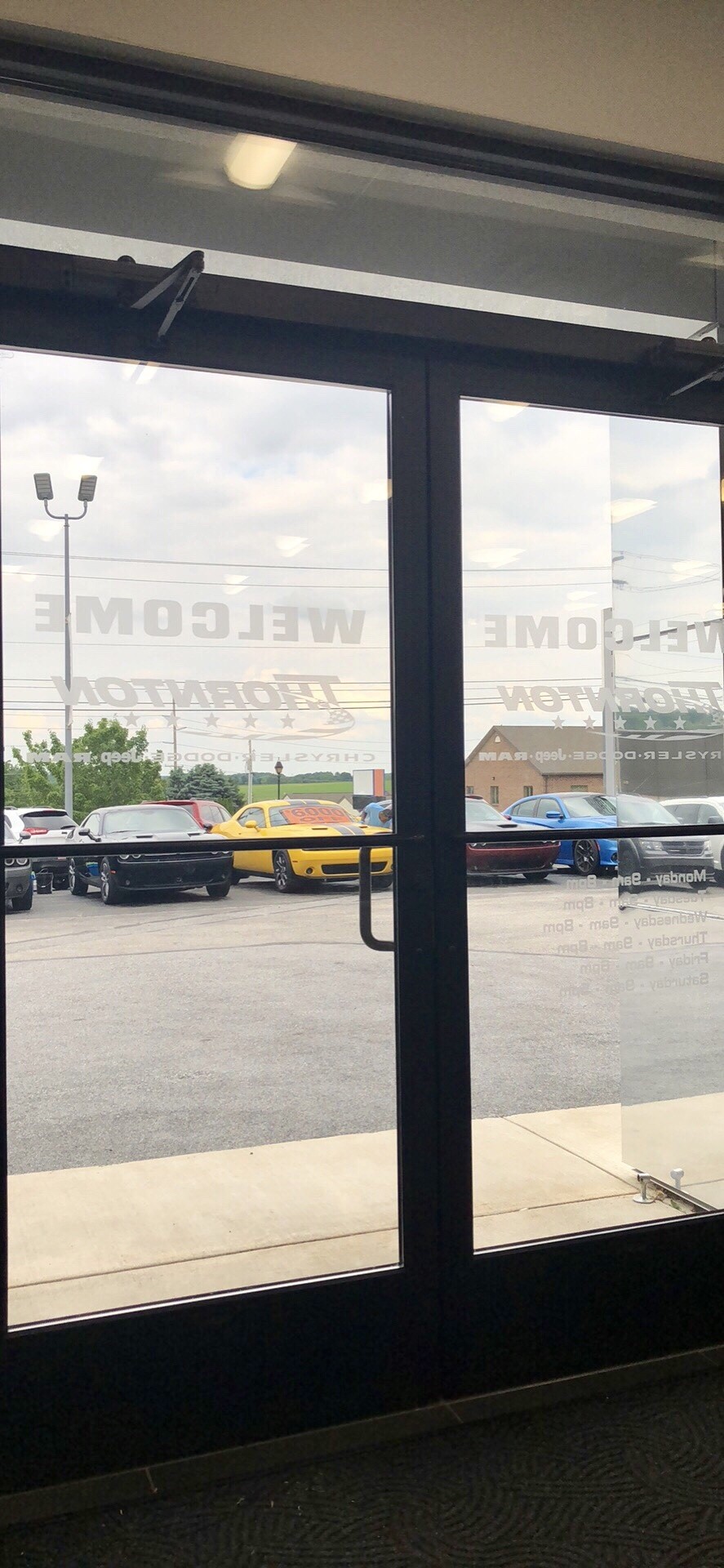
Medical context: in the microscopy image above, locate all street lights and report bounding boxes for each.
[273,761,284,800]
[34,472,98,821]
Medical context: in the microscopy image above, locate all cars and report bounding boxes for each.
[467,798,561,884]
[5,806,79,879]
[3,822,33,911]
[210,800,395,893]
[66,805,233,906]
[141,799,233,831]
[502,793,617,877]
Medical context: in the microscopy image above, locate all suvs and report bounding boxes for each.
[659,795,724,885]
[618,791,715,894]
